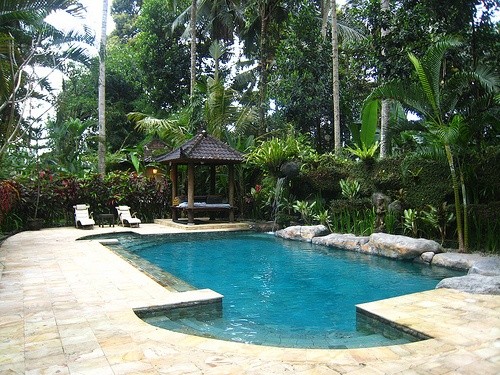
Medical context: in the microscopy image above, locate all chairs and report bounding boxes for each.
[73,203,96,230]
[115,205,140,227]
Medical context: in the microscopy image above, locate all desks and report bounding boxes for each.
[98,214,113,228]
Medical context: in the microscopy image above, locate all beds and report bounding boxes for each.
[171,201,232,210]
